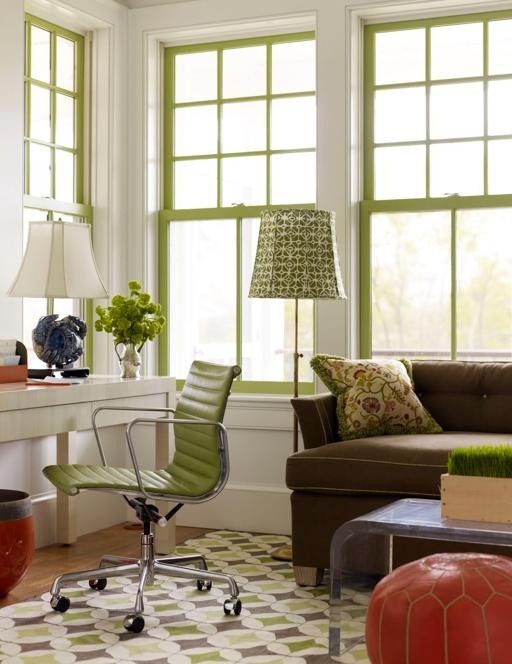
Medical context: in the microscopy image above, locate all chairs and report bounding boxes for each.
[44,360,242,633]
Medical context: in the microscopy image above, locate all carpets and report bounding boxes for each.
[1,529,385,664]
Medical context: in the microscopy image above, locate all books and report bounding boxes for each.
[0,339,21,367]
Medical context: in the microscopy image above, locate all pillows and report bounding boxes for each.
[307,353,445,442]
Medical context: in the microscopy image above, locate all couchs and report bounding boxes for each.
[284,361,512,588]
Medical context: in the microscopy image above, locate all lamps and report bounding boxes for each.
[7,216,109,369]
[248,210,347,562]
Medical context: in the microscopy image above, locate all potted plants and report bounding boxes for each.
[439,445,512,525]
[94,277,167,376]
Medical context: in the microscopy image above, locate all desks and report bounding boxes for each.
[0,375,177,557]
